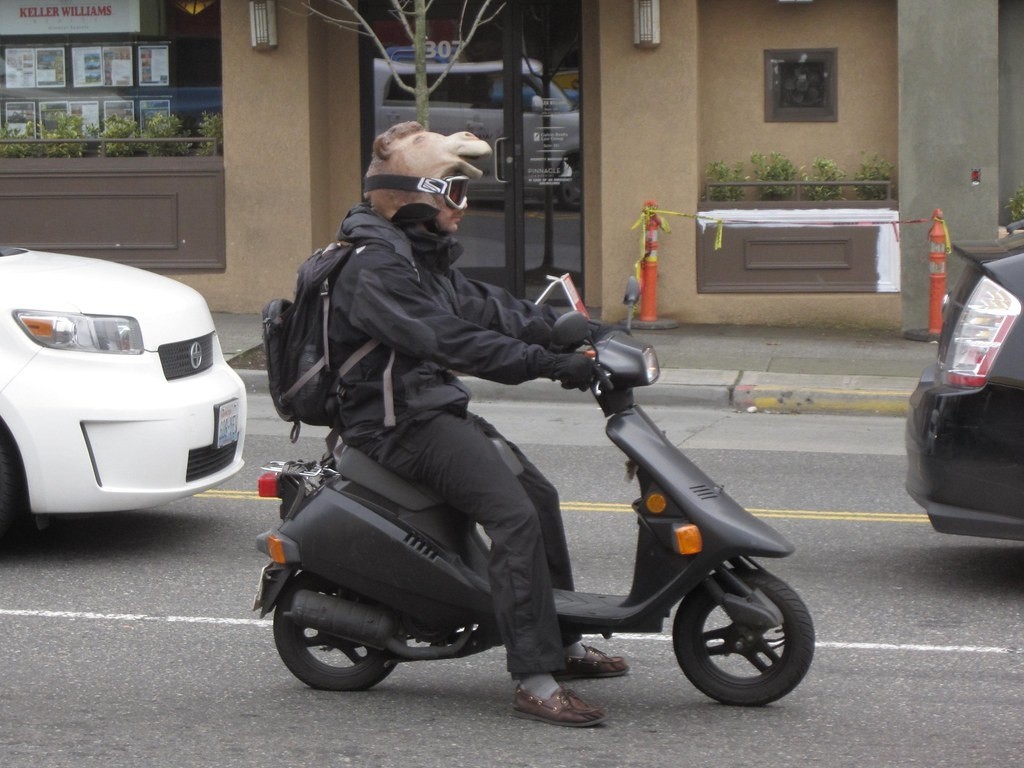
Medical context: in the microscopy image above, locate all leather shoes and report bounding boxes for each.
[513,683,609,726]
[551,643,629,681]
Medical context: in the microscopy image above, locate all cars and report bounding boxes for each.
[1,245,247,546]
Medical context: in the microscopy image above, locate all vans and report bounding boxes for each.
[373,57,581,212]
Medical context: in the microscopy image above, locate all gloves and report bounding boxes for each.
[536,353,614,392]
[583,320,631,345]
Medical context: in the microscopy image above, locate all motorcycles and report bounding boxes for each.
[252,274,815,708]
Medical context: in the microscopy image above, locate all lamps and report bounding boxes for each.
[632,1,660,52]
[248,1,278,52]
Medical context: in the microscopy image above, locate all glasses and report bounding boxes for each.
[363,174,470,210]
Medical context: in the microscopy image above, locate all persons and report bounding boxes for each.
[331,121,631,727]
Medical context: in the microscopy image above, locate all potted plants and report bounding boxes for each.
[696,150,903,294]
[0,109,226,272]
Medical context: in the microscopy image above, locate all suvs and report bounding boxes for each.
[904,219,1023,541]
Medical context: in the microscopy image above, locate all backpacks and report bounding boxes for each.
[263,237,396,444]
